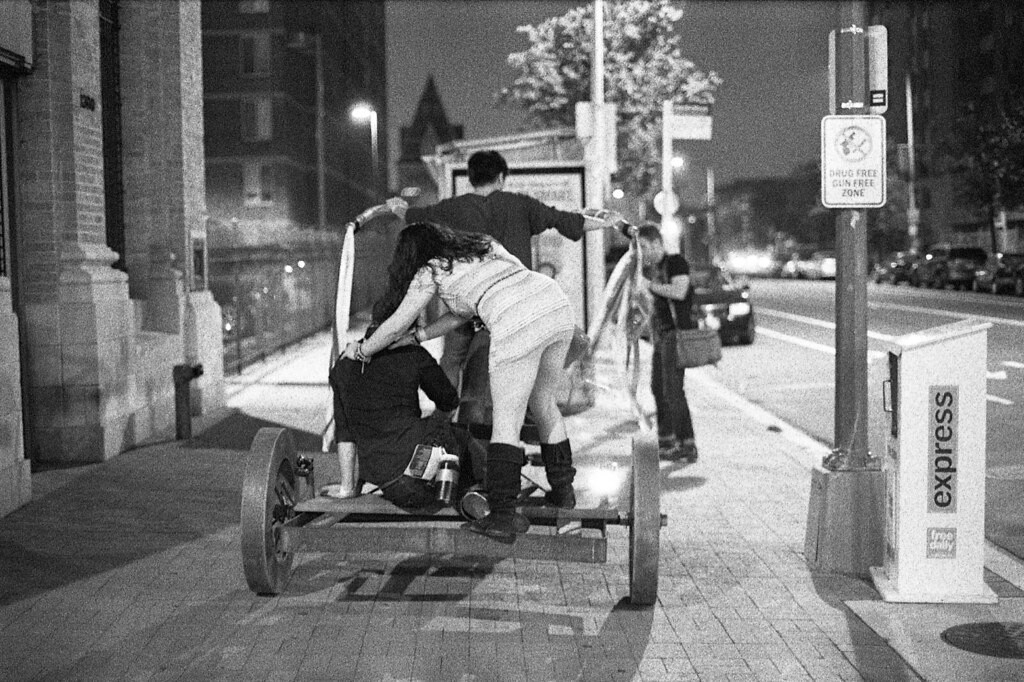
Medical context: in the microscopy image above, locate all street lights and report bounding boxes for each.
[351,107,379,208]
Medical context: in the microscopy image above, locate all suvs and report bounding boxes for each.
[908,242,987,290]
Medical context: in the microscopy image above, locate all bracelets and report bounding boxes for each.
[354,343,371,373]
[391,204,408,213]
[417,326,427,342]
[386,149,625,425]
[408,326,421,344]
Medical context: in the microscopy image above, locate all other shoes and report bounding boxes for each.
[659,444,697,464]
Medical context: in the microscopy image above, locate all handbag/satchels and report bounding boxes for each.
[675,328,722,368]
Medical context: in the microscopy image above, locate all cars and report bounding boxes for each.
[971,251,1024,295]
[872,251,923,286]
[630,261,755,345]
[1012,262,1024,298]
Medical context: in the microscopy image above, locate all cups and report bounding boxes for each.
[433,453,461,508]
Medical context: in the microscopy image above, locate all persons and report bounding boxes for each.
[320,292,531,533]
[633,222,698,463]
[338,218,576,544]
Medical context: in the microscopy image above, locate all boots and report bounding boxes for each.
[460,444,524,544]
[540,438,577,509]
